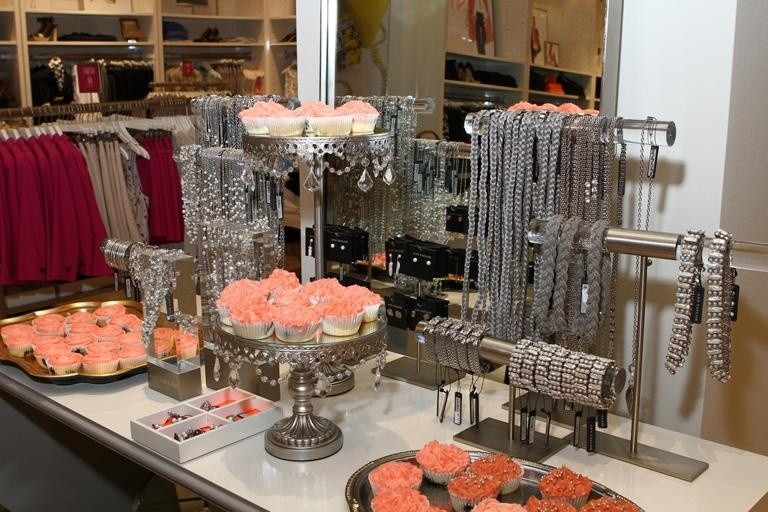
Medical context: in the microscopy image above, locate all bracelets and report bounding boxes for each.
[103,235,140,275]
[422,313,620,459]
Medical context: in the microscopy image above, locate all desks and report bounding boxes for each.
[0,291,768,512]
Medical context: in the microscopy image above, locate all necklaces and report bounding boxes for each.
[126,243,186,352]
[171,94,739,382]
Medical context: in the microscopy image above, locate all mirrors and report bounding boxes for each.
[315,0,624,358]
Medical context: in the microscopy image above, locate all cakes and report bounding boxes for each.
[238,98,381,118]
[368,439,642,512]
[215,269,382,327]
[0,303,198,366]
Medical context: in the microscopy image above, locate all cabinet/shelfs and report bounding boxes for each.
[0,0,297,129]
[384,0,604,141]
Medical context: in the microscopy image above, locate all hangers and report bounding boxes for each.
[0,58,245,163]
